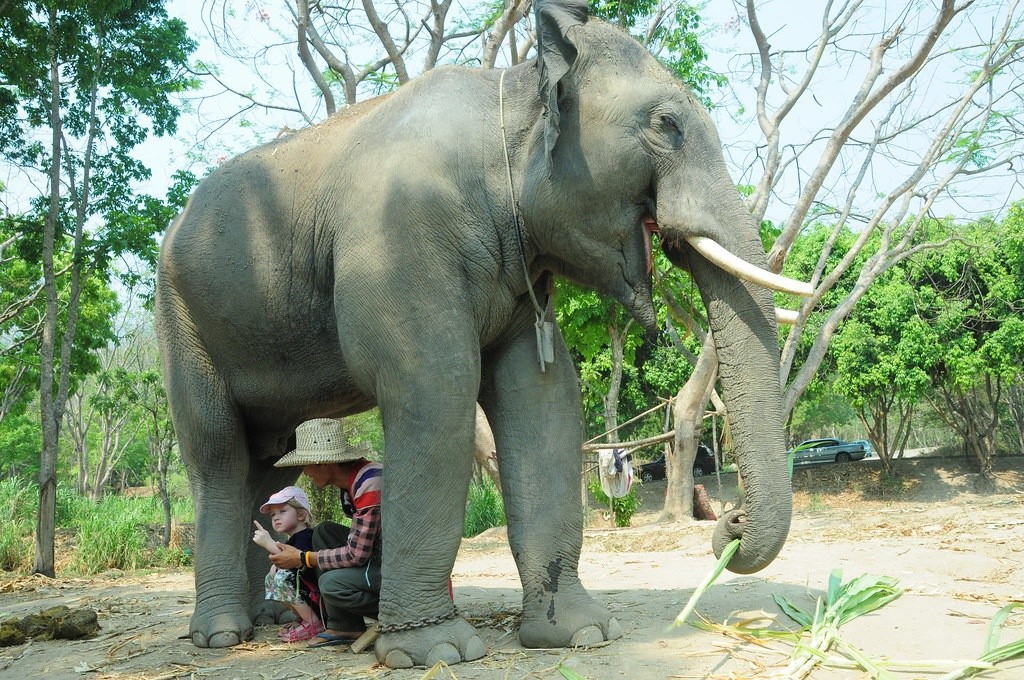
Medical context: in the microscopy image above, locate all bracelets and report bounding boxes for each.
[300,551,313,568]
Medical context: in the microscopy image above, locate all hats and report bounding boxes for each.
[273,417,369,467]
[260,486,311,517]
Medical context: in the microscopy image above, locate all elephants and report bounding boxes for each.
[152,0,816,667]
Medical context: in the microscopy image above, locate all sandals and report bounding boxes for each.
[278,620,326,642]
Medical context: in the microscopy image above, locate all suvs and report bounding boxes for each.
[636,444,723,483]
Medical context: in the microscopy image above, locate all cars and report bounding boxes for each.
[852,440,872,458]
[787,437,867,463]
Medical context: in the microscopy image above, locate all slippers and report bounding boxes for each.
[305,629,355,647]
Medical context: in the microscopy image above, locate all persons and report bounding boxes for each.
[268,418,383,648]
[252,486,325,643]
